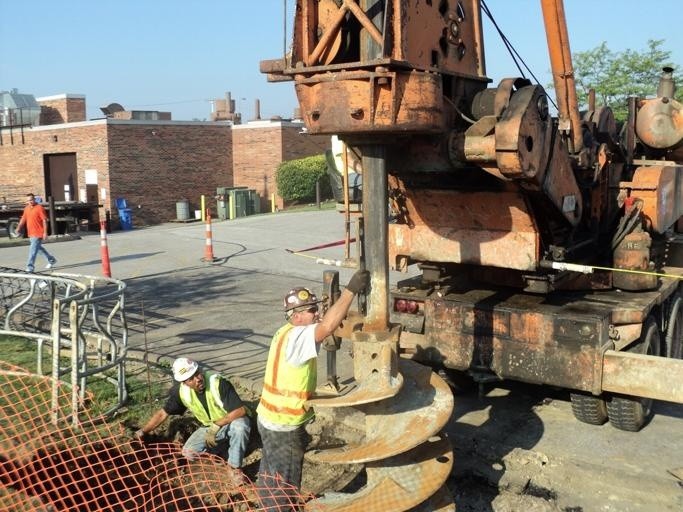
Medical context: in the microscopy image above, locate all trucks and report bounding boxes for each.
[0,196,103,240]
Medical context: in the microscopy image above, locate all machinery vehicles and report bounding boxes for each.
[260,0,683,512]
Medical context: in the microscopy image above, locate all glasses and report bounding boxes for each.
[298,306,319,314]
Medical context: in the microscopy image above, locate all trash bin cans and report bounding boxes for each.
[115,198,132,230]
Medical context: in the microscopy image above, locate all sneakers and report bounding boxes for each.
[225,463,248,489]
[44,258,57,269]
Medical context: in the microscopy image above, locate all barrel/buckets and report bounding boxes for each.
[195,210,201,219]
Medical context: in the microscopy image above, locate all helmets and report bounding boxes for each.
[169,356,200,383]
[281,285,325,313]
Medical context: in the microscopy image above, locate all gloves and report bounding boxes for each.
[344,268,371,296]
[130,429,148,442]
[203,420,222,450]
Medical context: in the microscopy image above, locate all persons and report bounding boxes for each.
[254,266,372,511]
[14,193,56,272]
[131,358,254,486]
